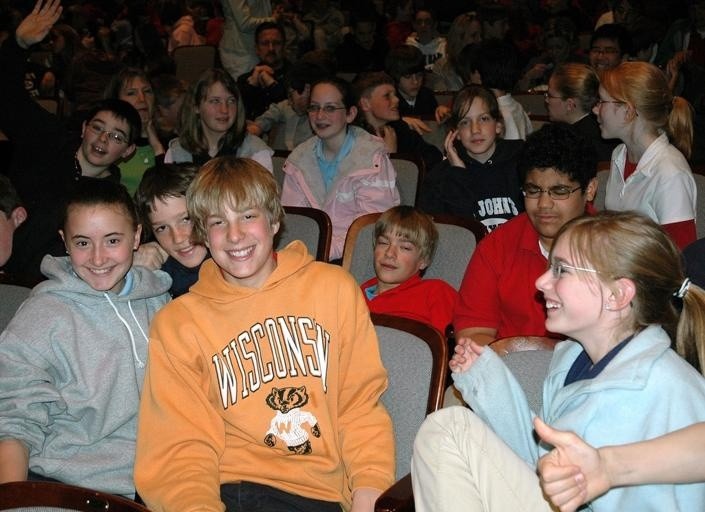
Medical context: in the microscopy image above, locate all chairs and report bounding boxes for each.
[0,43,704,512]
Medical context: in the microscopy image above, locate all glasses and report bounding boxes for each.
[520,184,582,200]
[87,123,129,144]
[547,261,613,279]
[306,103,346,113]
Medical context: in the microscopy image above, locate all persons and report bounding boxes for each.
[1,2,704,408]
[2,184,174,501]
[409,210,705,512]
[137,156,395,511]
